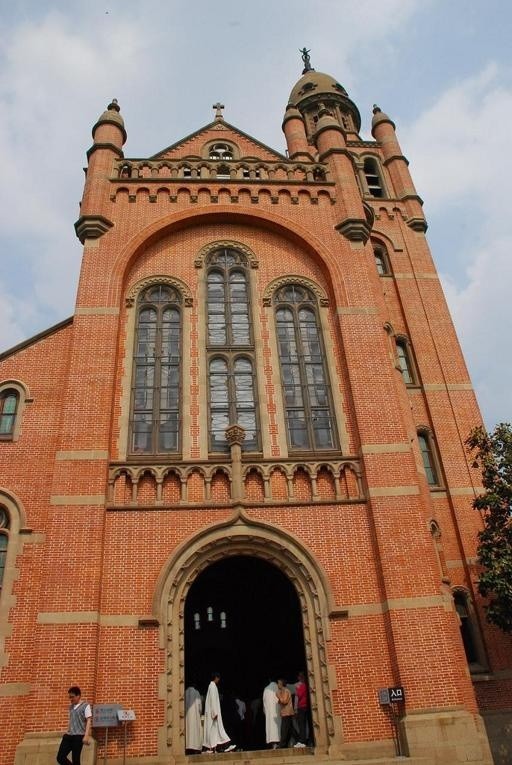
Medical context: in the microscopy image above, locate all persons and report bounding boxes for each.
[184,671,314,755]
[56,685,93,765]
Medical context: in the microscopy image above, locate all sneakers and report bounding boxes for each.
[294,742,306,748]
[224,744,236,752]
[201,750,213,754]
